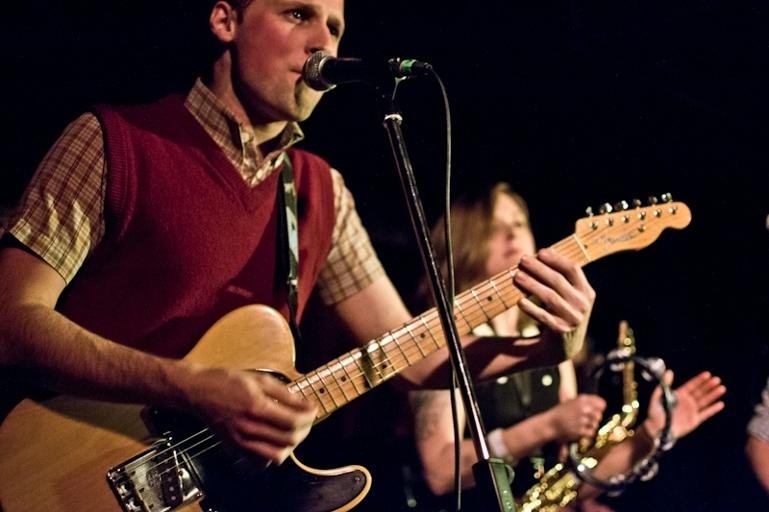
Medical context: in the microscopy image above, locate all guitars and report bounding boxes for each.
[0,192,692,511]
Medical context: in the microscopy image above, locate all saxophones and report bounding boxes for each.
[517,322,641,512]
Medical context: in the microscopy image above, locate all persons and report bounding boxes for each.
[0,0,598,466]
[407,183,728,512]
[745,380,769,491]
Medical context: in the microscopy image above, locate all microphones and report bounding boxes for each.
[301,51,431,92]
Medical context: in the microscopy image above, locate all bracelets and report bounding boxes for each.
[488,427,515,466]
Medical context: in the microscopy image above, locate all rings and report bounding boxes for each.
[586,416,593,429]
